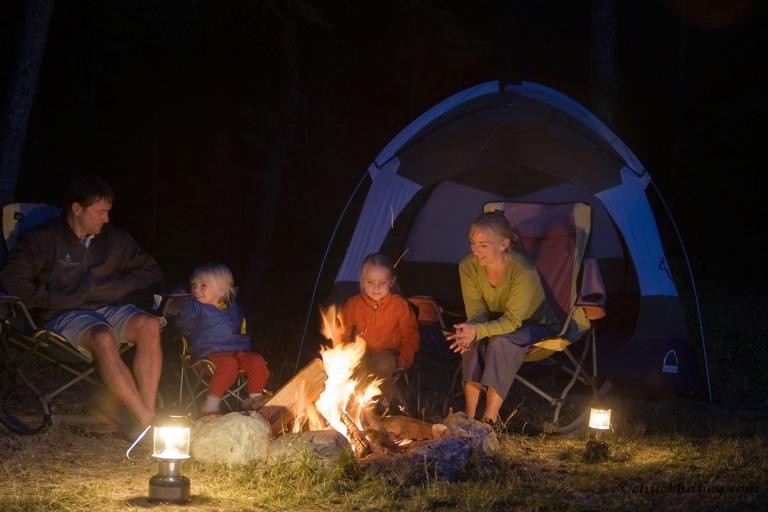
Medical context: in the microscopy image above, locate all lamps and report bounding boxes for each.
[583,396,614,465]
[126,410,192,504]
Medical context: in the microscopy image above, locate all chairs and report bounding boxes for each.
[445,203,613,431]
[401,292,457,421]
[0,202,174,435]
[163,291,270,421]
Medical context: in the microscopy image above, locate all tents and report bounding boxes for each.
[300,73,716,412]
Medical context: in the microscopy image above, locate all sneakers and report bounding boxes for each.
[127,412,170,445]
[479,414,501,433]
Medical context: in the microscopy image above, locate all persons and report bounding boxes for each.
[445,209,564,428]
[174,261,271,421]
[335,251,423,418]
[3,177,174,451]
